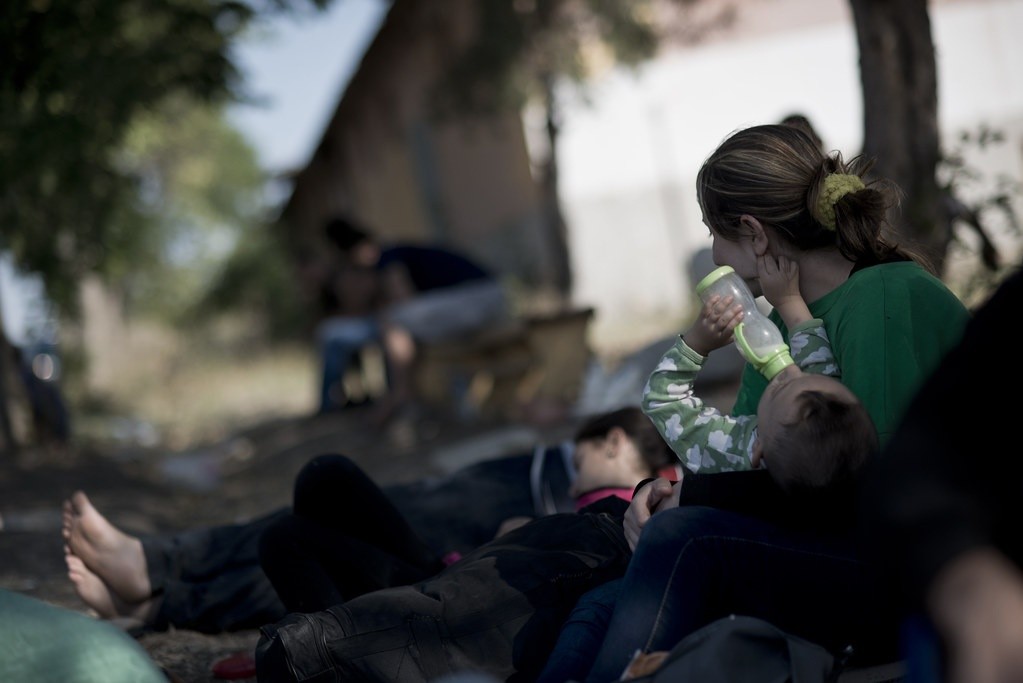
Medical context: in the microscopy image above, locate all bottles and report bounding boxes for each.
[695,266,794,380]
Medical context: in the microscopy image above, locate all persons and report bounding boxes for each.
[317,234,418,423]
[540,123,969,682]
[494,515,533,540]
[61,442,583,641]
[784,113,822,147]
[573,407,686,524]
[637,259,1023,682]
[626,259,878,516]
